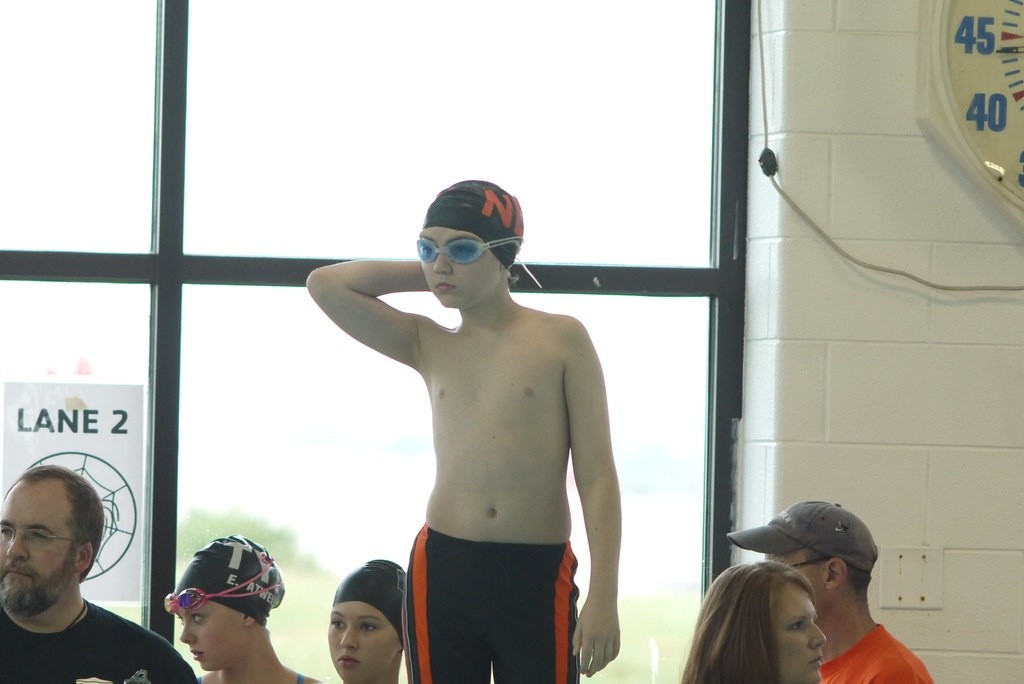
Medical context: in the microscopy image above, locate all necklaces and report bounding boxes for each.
[64,600,87,630]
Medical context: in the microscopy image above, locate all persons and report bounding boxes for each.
[306,178,622,684]
[164,534,325,684]
[328,560,407,684]
[0,465,198,684]
[726,501,935,684]
[682,561,827,684]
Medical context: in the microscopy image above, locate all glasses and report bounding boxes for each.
[164,587,205,615]
[0,523,85,549]
[416,236,526,264]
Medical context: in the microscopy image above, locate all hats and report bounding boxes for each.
[174,534,285,627]
[728,500,879,572]
[423,179,524,270]
[332,559,407,648]
